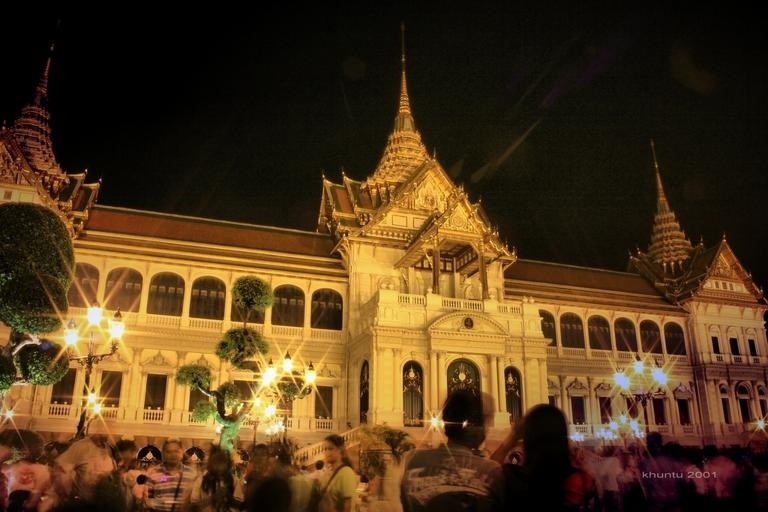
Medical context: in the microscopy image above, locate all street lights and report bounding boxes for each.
[565,352,673,453]
[81,388,102,440]
[236,396,278,451]
[261,348,318,443]
[64,295,126,444]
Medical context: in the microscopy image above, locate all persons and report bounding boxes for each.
[1,429,359,512]
[402,390,767,512]
